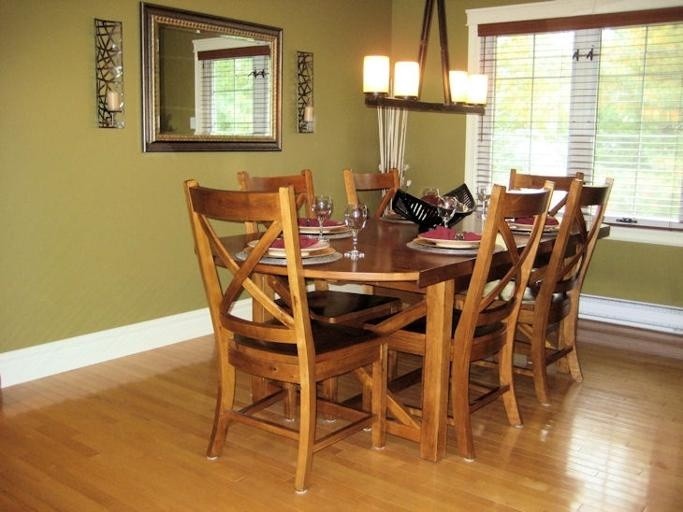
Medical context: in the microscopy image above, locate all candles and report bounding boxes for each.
[106,91,122,110]
[302,106,315,122]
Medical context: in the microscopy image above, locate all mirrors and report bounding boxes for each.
[140,2,283,153]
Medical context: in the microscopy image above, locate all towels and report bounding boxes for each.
[514,216,559,225]
[422,225,481,241]
[269,235,316,249]
[297,217,337,227]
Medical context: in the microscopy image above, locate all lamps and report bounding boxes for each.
[359,3,490,117]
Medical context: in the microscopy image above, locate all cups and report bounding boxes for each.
[314,197,333,241]
[476,184,491,219]
[437,197,457,229]
[344,204,365,259]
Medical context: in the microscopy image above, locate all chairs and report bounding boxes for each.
[458,176,615,407]
[507,168,586,243]
[232,170,400,432]
[183,178,392,494]
[339,167,400,220]
[361,181,557,462]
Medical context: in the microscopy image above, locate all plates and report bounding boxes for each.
[248,240,328,257]
[511,224,559,232]
[406,241,502,255]
[235,250,343,265]
[419,233,481,248]
[243,248,336,257]
[299,221,346,233]
